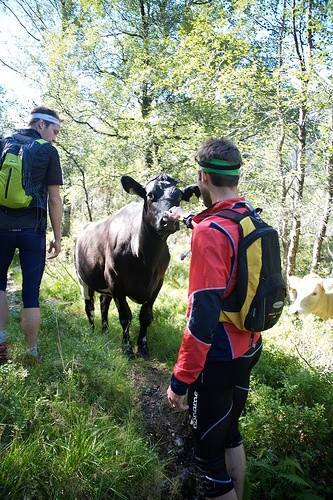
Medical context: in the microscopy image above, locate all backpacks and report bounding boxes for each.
[0,132,50,210]
[199,200,287,333]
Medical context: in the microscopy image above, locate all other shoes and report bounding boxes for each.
[0,341,9,364]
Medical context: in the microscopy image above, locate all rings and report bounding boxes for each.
[173,404,176,408]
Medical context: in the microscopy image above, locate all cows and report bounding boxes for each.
[72,169,202,362]
[282,270,333,320]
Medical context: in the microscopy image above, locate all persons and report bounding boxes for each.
[165,137,264,500]
[0,106,63,361]
